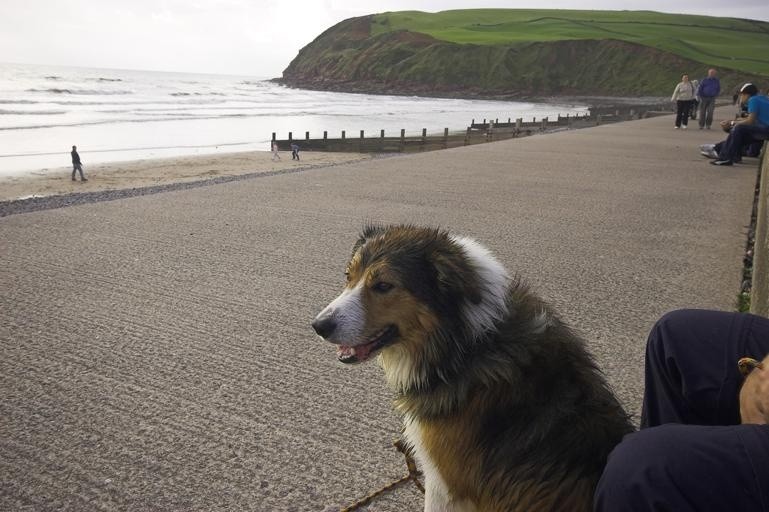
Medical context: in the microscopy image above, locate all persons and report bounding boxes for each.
[290,141,302,160]
[595,307,768,509]
[272,140,281,162]
[669,66,769,168]
[69,145,86,184]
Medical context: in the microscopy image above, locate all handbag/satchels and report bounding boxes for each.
[745,137,764,158]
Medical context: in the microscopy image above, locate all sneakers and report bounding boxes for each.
[700,151,720,159]
[710,158,733,166]
[697,144,716,151]
[673,125,711,129]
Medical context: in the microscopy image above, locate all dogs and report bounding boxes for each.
[312,218,640,512]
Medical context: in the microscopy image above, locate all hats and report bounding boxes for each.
[737,83,757,94]
